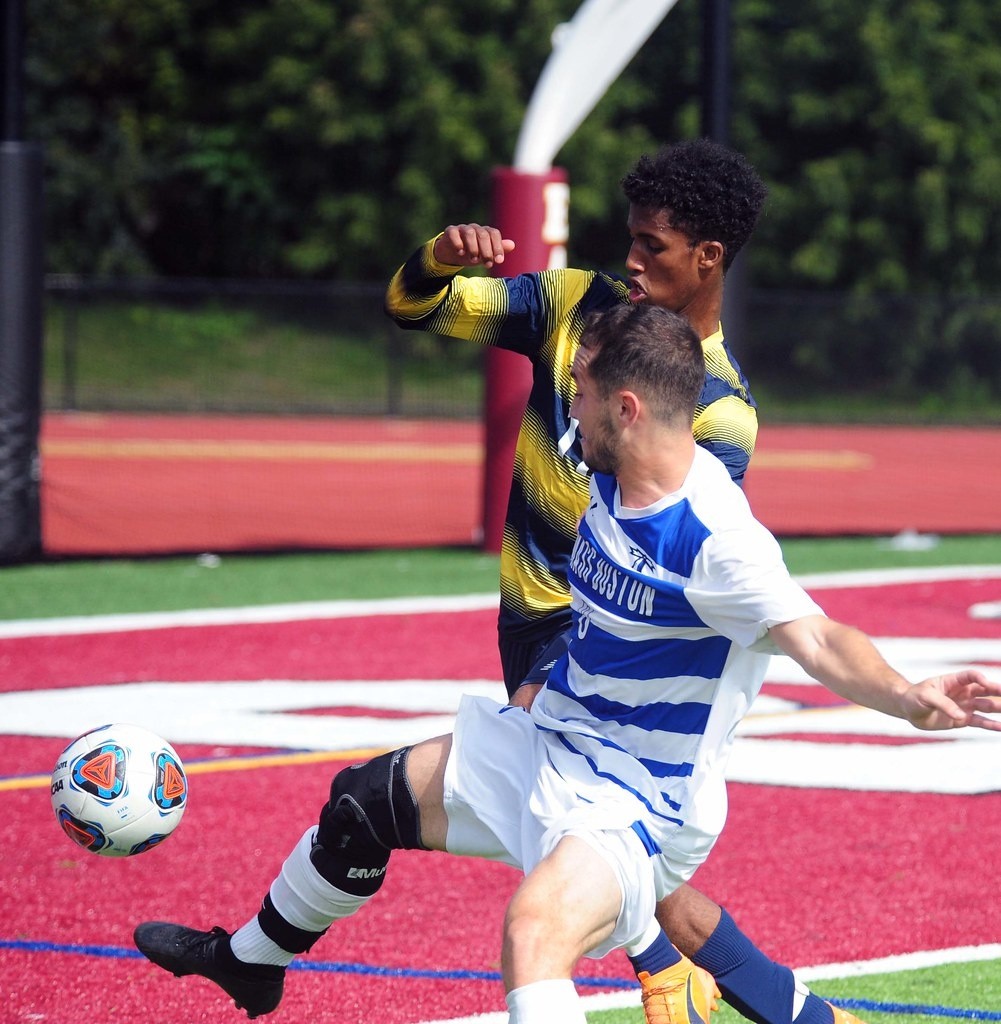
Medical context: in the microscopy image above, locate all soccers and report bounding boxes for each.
[51,720,188,858]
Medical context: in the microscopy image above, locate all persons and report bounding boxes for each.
[383,136,869,1024]
[132,300,1001,1024]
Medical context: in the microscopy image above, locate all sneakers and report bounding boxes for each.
[637,944,722,1024]
[134,921,285,1019]
[825,1001,866,1024]
